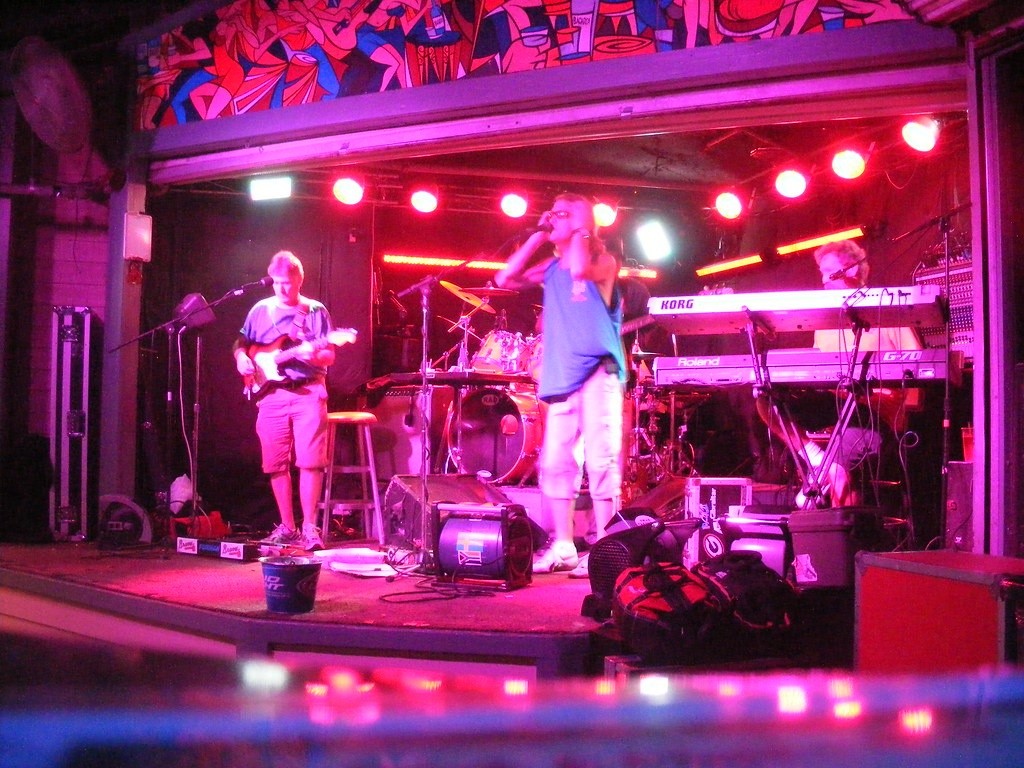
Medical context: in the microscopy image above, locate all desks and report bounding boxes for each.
[385,372,538,487]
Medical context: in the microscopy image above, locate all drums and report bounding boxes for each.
[528,333,544,383]
[472,329,526,373]
[446,386,547,486]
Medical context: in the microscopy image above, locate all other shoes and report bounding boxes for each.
[568,552,589,578]
[533,545,577,572]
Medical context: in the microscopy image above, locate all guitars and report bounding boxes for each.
[239,327,359,401]
[621,282,735,335]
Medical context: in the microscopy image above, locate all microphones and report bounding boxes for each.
[242,277,274,289]
[524,222,554,233]
[829,271,847,280]
[404,398,414,426]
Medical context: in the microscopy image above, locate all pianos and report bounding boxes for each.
[645,282,966,514]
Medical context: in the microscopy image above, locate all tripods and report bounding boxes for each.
[106,288,249,558]
[623,329,699,502]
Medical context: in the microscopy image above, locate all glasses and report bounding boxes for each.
[547,211,572,220]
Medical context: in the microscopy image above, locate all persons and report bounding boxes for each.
[234,252,334,550]
[497,194,626,578]
[755,242,925,508]
[579,234,714,542]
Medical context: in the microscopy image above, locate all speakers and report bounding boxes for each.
[385,472,548,555]
[357,385,457,481]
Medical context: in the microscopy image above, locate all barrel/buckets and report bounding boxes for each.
[258,556,322,615]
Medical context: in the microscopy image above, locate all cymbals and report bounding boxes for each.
[631,351,665,359]
[439,280,497,314]
[458,287,520,298]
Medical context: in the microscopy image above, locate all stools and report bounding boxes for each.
[313,412,386,546]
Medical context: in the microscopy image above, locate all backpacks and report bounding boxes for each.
[613,543,797,664]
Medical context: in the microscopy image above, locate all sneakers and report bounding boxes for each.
[261,523,301,544]
[301,523,324,551]
